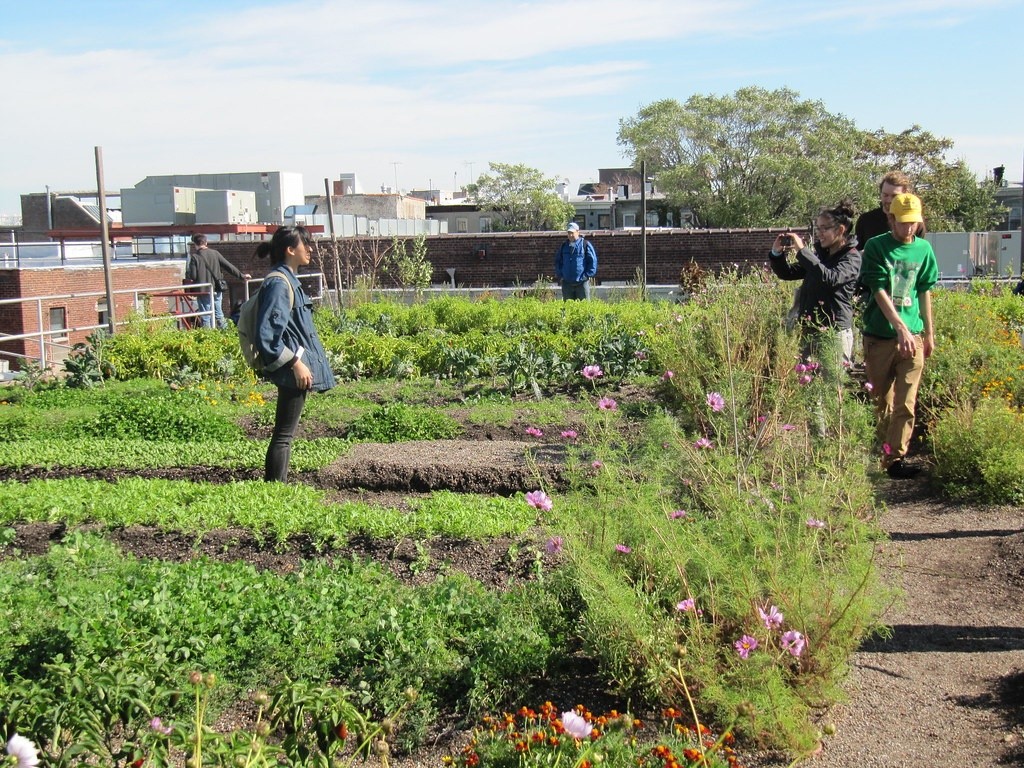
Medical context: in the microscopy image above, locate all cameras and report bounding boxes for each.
[779,236,792,247]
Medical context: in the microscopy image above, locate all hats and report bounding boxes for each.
[567,222,579,232]
[889,193,924,223]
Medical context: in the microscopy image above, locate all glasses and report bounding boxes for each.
[814,225,837,233]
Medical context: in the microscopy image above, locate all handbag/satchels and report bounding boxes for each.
[788,285,803,321]
[215,279,228,292]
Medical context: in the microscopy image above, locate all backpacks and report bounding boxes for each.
[237,273,294,370]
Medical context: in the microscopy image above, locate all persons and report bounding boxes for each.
[188,234,252,329]
[847,171,926,374]
[768,197,862,440]
[254,224,336,482]
[554,223,598,302]
[859,194,938,479]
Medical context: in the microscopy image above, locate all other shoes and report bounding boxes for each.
[887,459,907,480]
